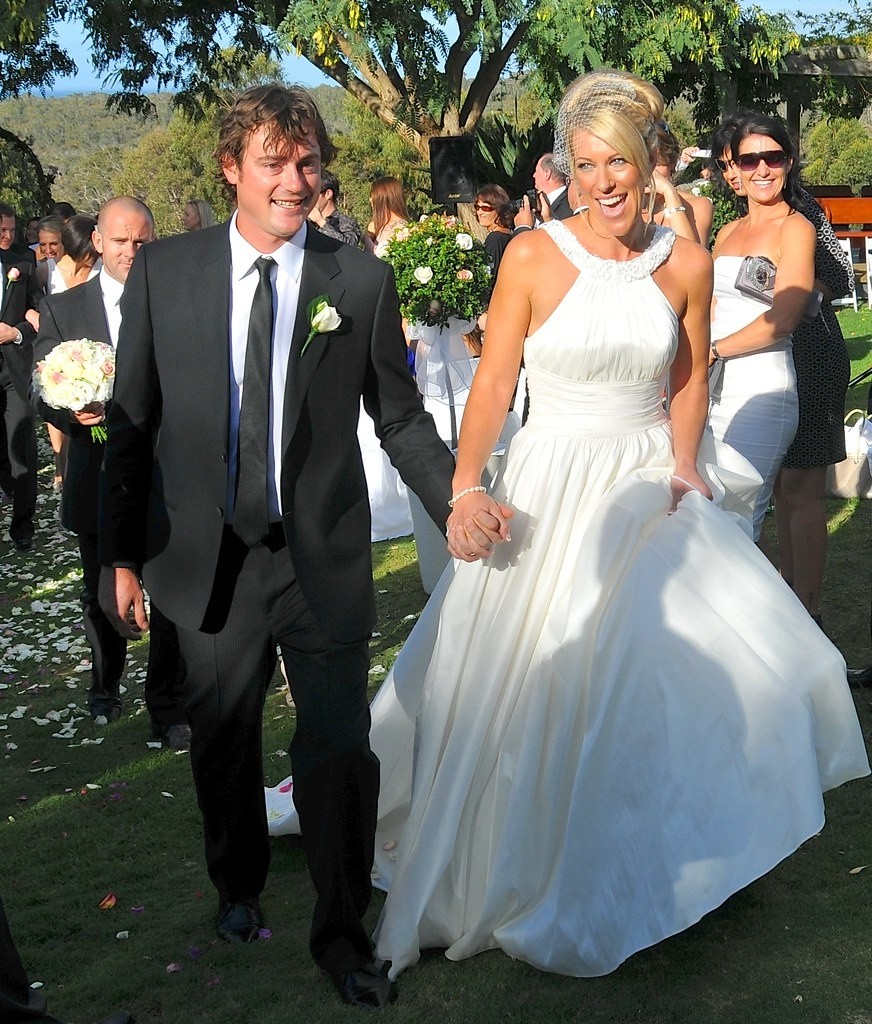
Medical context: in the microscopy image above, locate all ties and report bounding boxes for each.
[232,256,276,546]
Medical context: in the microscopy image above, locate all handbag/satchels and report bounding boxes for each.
[736,256,824,324]
[822,408,872,499]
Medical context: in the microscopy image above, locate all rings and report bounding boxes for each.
[470,553,475,556]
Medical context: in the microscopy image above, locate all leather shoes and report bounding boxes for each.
[217,894,263,947]
[330,964,397,1013]
[850,663,872,688]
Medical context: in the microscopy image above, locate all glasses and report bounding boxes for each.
[474,204,497,212]
[654,120,670,136]
[734,150,788,171]
[566,176,574,187]
[714,159,737,173]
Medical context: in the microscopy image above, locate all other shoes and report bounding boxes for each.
[52,471,63,491]
[92,1009,136,1024]
[14,536,34,552]
[93,705,120,724]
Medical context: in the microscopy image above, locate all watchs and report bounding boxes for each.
[15,332,21,342]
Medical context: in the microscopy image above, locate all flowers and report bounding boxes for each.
[300,295,343,357]
[33,338,116,444]
[381,212,495,336]
[6,267,21,289]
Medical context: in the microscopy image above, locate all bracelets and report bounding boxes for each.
[665,206,686,214]
[447,486,486,507]
[712,340,720,359]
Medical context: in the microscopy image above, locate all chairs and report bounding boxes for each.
[830,236,872,313]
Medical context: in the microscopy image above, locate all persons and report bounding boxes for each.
[711,110,855,633]
[672,143,715,182]
[264,67,872,981]
[642,118,714,250]
[0,197,102,552]
[100,85,506,999]
[360,175,418,381]
[303,168,361,250]
[181,198,215,233]
[474,153,590,332]
[704,114,818,551]
[32,196,192,755]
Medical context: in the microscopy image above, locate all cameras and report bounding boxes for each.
[509,189,542,214]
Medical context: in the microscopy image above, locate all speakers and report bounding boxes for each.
[429,135,478,203]
